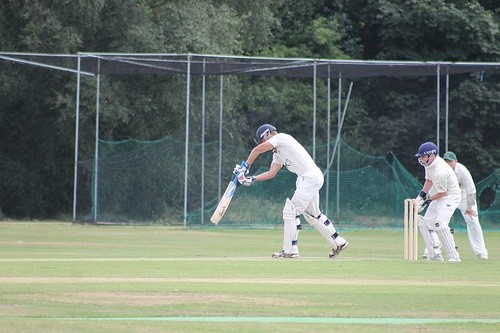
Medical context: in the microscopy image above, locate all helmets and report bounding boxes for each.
[415,141,439,166]
[254,124,277,144]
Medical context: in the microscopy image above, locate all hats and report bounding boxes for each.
[443,151,457,161]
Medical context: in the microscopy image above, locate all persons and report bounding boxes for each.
[414,142,461,262]
[233,124,349,258]
[422,152,488,259]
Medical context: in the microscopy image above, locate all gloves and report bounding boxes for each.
[233,161,251,176]
[238,173,256,187]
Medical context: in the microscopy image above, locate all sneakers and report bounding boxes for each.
[272,250,300,258]
[328,241,350,258]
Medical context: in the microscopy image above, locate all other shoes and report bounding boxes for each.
[448,257,462,261]
[431,255,445,260]
[423,254,428,259]
